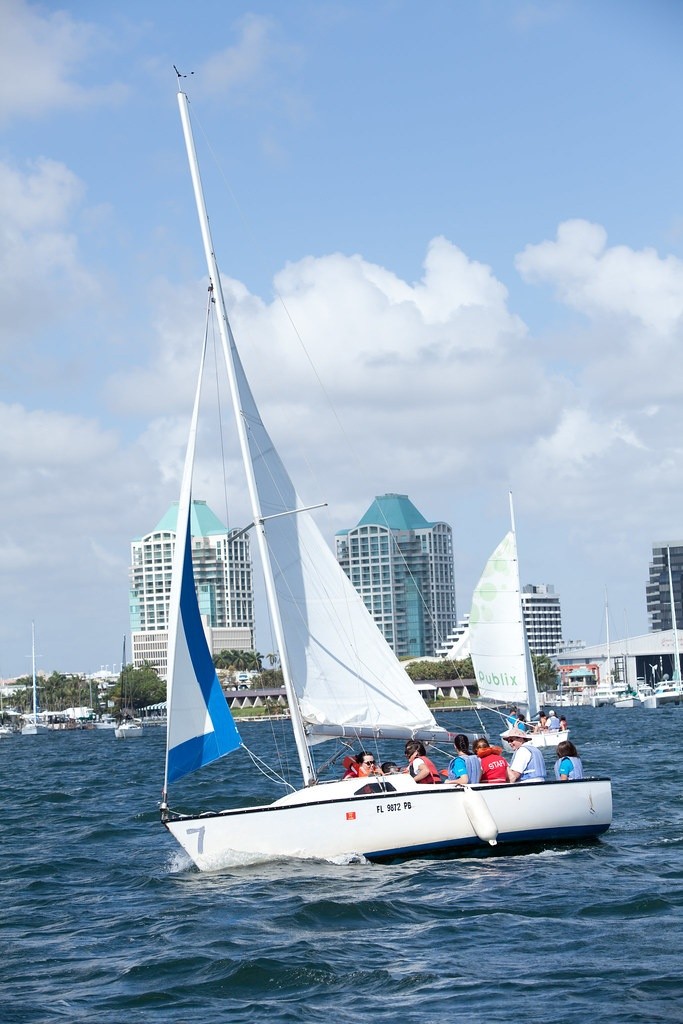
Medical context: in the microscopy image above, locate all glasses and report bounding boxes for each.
[513,711,516,713]
[476,744,487,749]
[508,738,517,743]
[363,760,375,766]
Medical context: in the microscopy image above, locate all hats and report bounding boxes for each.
[549,710,554,715]
[500,728,532,741]
[538,711,545,716]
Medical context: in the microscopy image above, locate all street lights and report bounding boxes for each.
[113,664,116,674]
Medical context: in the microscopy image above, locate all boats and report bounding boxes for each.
[40,705,118,731]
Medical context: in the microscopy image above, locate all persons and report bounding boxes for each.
[445,734,481,784]
[343,751,383,779]
[538,710,566,732]
[507,708,527,731]
[500,727,547,782]
[472,738,511,783]
[380,762,400,775]
[405,740,442,784]
[554,741,584,780]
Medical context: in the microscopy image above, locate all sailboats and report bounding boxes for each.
[114,634,143,738]
[539,543,682,709]
[22,622,48,734]
[157,62,613,874]
[469,490,571,753]
[0,676,14,739]
